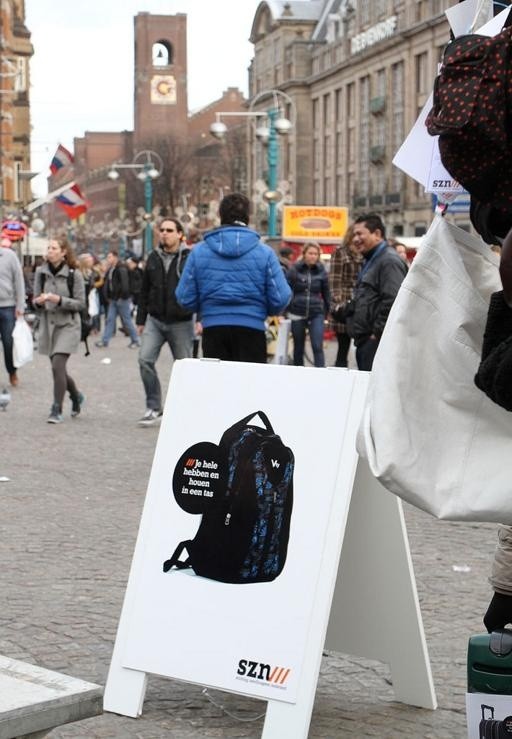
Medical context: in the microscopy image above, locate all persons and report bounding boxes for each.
[174,194,292,363]
[341,218,408,370]
[434,2,511,655]
[32,234,88,425]
[324,223,365,366]
[0,237,23,388]
[136,217,204,429]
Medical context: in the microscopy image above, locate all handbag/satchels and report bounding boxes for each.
[9,318,36,369]
[362,177,512,524]
[275,316,292,367]
[466,624,512,738]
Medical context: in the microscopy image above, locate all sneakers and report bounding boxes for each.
[67,392,85,418]
[138,408,164,427]
[10,373,18,387]
[96,339,107,348]
[126,341,141,350]
[47,404,63,423]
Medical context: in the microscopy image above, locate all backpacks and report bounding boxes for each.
[40,267,94,342]
[114,265,144,296]
[159,409,298,584]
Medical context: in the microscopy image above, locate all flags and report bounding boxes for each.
[48,143,77,175]
[55,184,93,217]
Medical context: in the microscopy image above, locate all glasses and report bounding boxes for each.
[158,227,180,233]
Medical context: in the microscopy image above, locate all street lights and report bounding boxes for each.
[106,149,165,256]
[209,88,297,237]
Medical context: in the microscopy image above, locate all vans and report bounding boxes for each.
[21,239,50,270]
